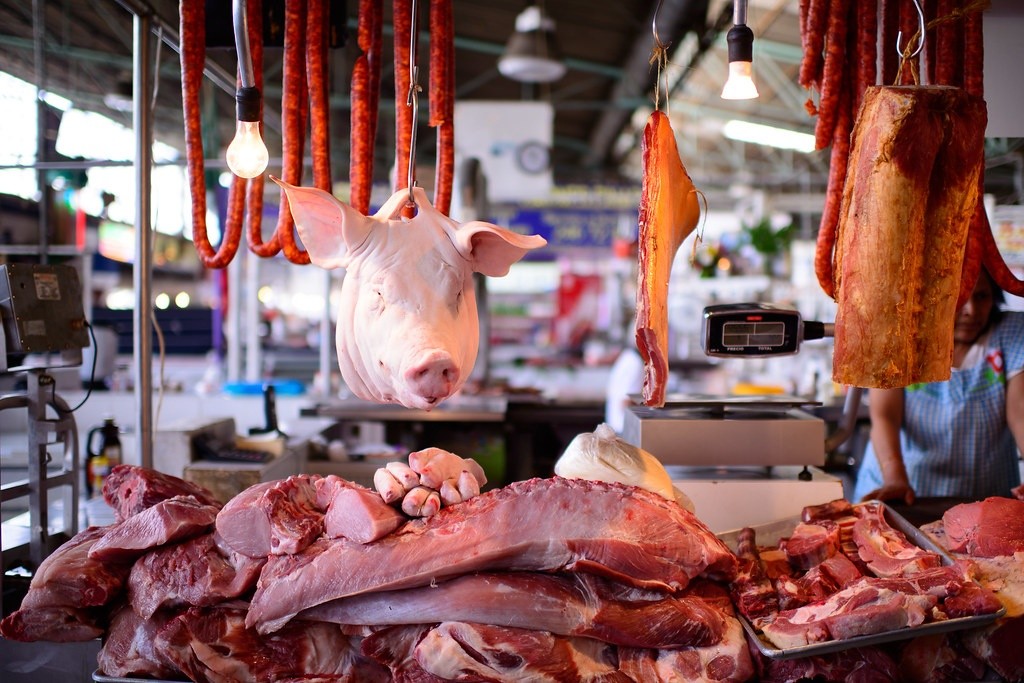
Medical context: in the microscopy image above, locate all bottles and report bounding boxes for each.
[83,419,122,499]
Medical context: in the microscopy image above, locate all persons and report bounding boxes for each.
[83,326,118,391]
[606,321,646,450]
[853,273,1024,505]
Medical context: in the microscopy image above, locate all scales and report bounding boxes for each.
[0,262,98,573]
[607,302,864,481]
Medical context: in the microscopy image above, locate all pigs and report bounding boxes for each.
[266,172,548,410]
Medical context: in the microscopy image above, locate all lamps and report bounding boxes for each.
[496,6,568,80]
